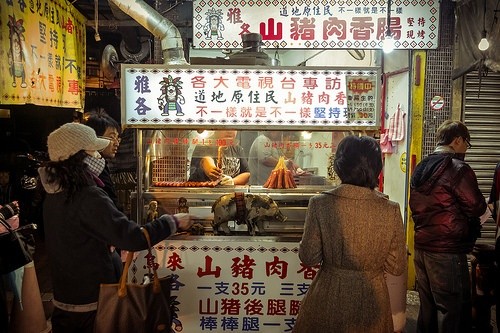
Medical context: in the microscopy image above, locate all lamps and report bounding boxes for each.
[478,0,489,51]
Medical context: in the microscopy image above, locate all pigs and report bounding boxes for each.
[210,192,288,236]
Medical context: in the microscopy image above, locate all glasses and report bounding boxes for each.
[98,137,121,144]
[460,135,472,149]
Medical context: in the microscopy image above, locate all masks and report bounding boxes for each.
[83,156,105,177]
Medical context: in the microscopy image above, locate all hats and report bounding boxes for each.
[48,123,111,161]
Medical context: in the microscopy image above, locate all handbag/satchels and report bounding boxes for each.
[0,218,37,276]
[94,227,170,333]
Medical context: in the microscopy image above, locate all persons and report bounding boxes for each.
[38,122,190,333]
[489,163,500,333]
[247,130,299,186]
[0,171,15,204]
[86,108,124,216]
[291,137,408,333]
[409,121,488,333]
[149,129,189,183]
[187,130,250,186]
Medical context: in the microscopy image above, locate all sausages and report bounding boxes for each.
[264,168,297,189]
[155,181,218,187]
